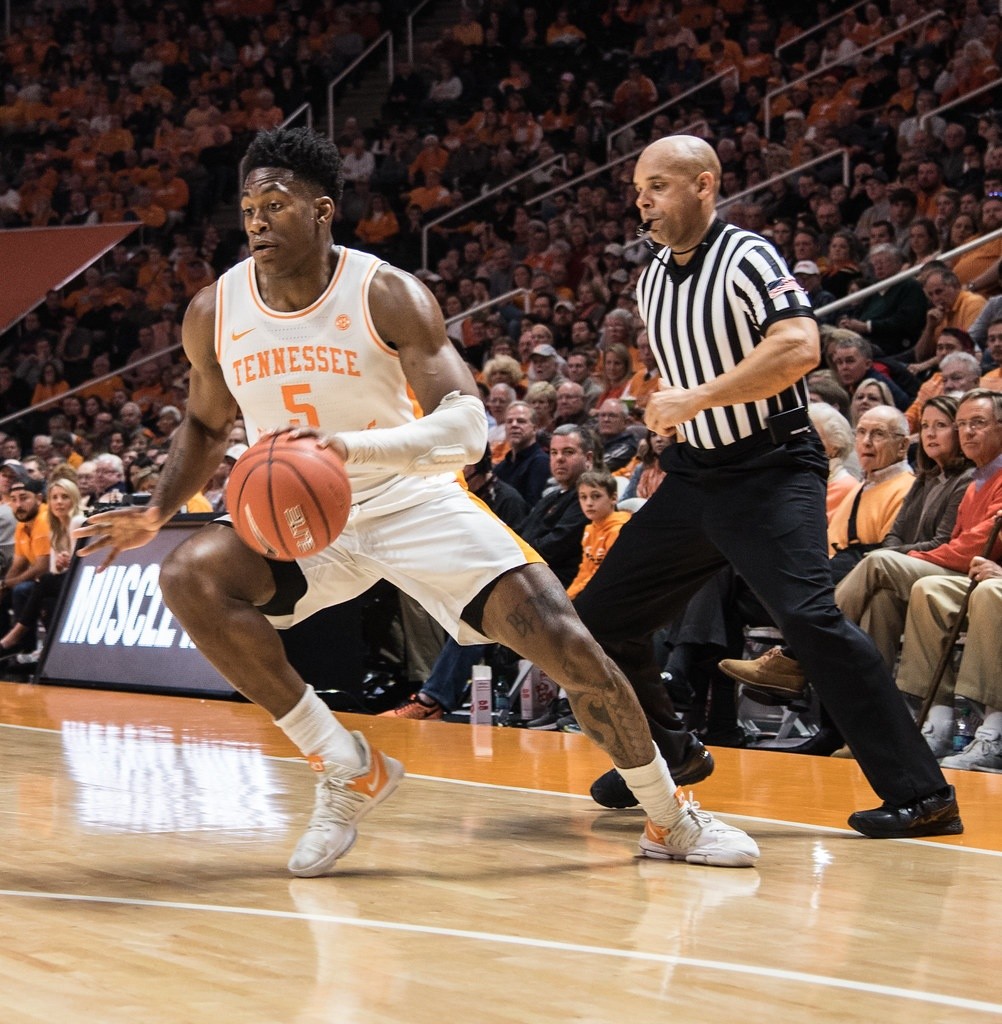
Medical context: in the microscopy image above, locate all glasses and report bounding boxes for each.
[853,428,903,442]
[951,418,999,430]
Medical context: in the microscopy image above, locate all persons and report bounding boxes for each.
[73,128,759,876]
[0,0,1002,772]
[570,135,965,839]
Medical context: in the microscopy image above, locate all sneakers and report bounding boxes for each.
[848,784,964,838]
[288,730,402,875]
[526,697,573,730]
[921,721,954,764]
[556,714,580,733]
[636,786,760,867]
[718,645,806,698]
[938,726,1002,774]
[589,743,714,809]
[378,694,443,720]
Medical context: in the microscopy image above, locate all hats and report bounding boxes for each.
[602,243,624,257]
[553,301,575,314]
[10,476,42,493]
[1,458,25,477]
[610,269,629,283]
[527,344,557,361]
[792,260,820,277]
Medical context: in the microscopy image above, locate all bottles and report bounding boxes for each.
[494,675,510,722]
[953,710,972,754]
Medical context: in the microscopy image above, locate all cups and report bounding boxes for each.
[622,400,637,409]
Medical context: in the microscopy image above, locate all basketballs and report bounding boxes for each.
[227,429,352,561]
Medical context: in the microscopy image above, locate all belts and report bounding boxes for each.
[680,430,773,467]
